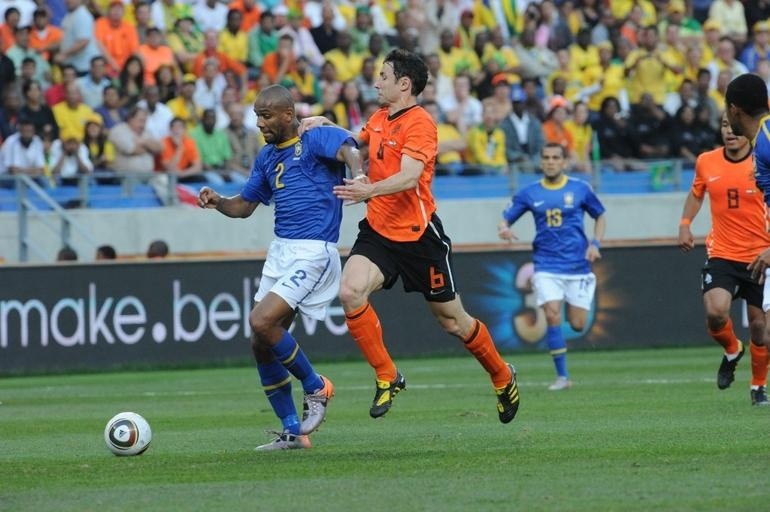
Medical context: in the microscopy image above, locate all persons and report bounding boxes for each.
[298,49,520,424]
[498,141,606,391]
[0,0,770,265]
[724,73,770,355]
[196,85,373,452]
[676,106,770,408]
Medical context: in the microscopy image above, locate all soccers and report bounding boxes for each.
[104,412,151,455]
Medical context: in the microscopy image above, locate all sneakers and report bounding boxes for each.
[548,375,572,390]
[716,338,745,390]
[254,431,311,452]
[298,375,336,435]
[369,370,406,418]
[749,383,769,408]
[489,362,520,424]
